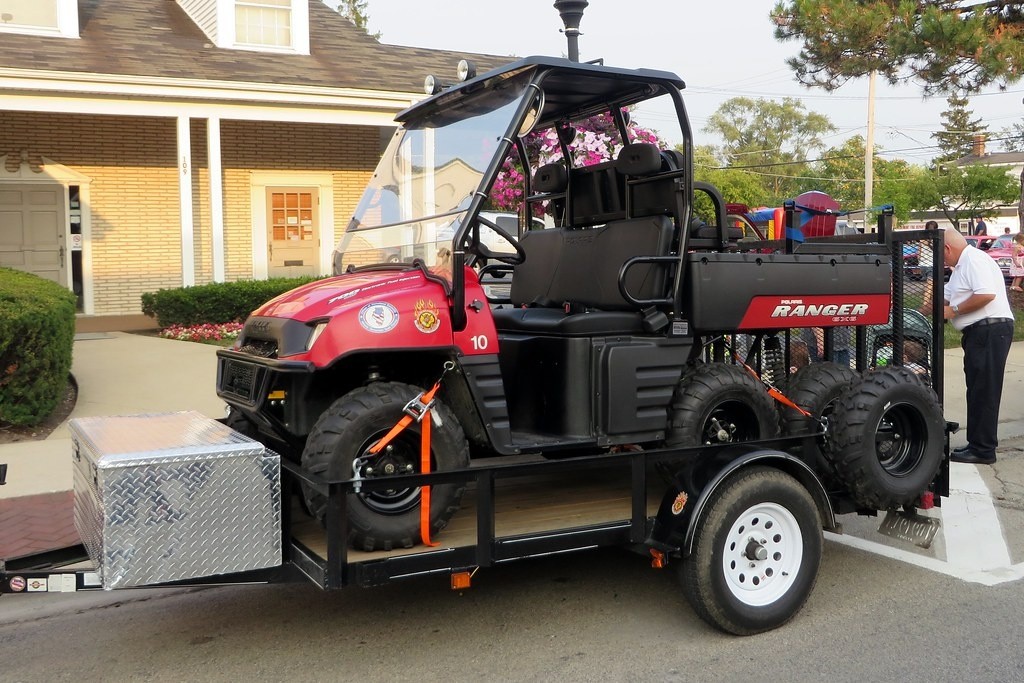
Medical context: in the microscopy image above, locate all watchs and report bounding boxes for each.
[951,304,962,315]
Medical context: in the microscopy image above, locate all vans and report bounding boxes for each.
[465,210,546,279]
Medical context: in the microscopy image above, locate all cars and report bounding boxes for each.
[944,236,998,280]
[903,243,924,281]
[984,233,1024,286]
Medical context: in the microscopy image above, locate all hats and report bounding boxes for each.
[973,214,982,218]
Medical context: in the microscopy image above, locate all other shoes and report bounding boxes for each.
[1009,285,1023,293]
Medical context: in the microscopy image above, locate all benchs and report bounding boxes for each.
[490,215,676,338]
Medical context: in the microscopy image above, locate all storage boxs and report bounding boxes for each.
[67,409,284,593]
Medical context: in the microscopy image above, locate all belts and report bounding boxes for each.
[960,314,1017,332]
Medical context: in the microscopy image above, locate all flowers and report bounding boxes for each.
[159,322,245,343]
[484,105,674,218]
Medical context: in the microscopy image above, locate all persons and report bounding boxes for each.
[902,340,960,430]
[920,228,1016,465]
[917,222,938,311]
[1003,227,1010,235]
[974,215,987,236]
[1007,232,1024,291]
[801,324,851,369]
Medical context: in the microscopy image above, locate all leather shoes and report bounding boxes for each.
[951,445,998,465]
